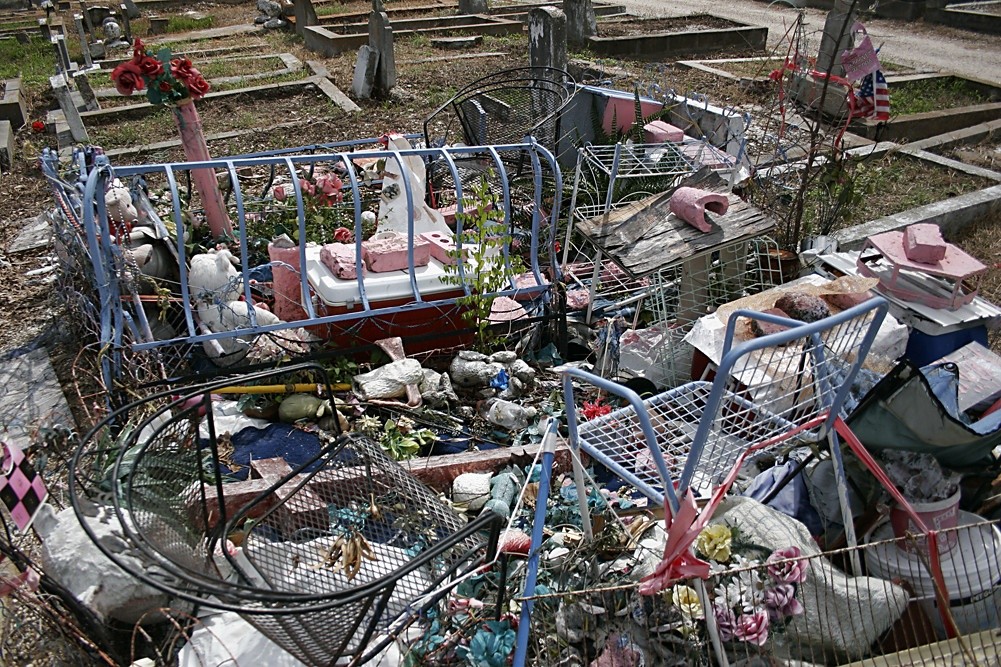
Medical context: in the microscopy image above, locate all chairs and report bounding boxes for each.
[423,66,575,251]
[68,361,508,666]
[562,296,889,667]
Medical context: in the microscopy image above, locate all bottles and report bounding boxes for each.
[476,396,529,430]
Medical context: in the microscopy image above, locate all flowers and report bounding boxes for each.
[579,316,615,421]
[406,572,515,667]
[248,172,350,247]
[657,523,810,646]
[112,38,209,128]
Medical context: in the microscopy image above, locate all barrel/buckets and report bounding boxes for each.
[863,510,1001,639]
[762,248,799,285]
[888,482,961,564]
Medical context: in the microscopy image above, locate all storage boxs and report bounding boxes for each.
[680,275,908,442]
[874,308,989,402]
[305,242,491,370]
[557,82,663,167]
